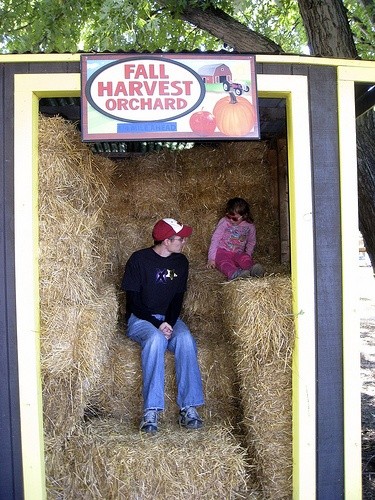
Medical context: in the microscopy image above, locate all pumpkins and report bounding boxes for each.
[213,92,254,134]
[189,107,217,136]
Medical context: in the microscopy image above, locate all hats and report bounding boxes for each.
[151,218,193,241]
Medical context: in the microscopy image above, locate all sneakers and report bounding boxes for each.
[178,406,204,429]
[139,408,158,433]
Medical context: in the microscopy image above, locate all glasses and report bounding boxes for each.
[225,212,245,222]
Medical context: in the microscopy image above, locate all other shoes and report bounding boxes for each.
[230,269,251,280]
[249,264,263,278]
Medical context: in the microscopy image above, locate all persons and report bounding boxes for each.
[206,198,264,281]
[120,217,206,433]
